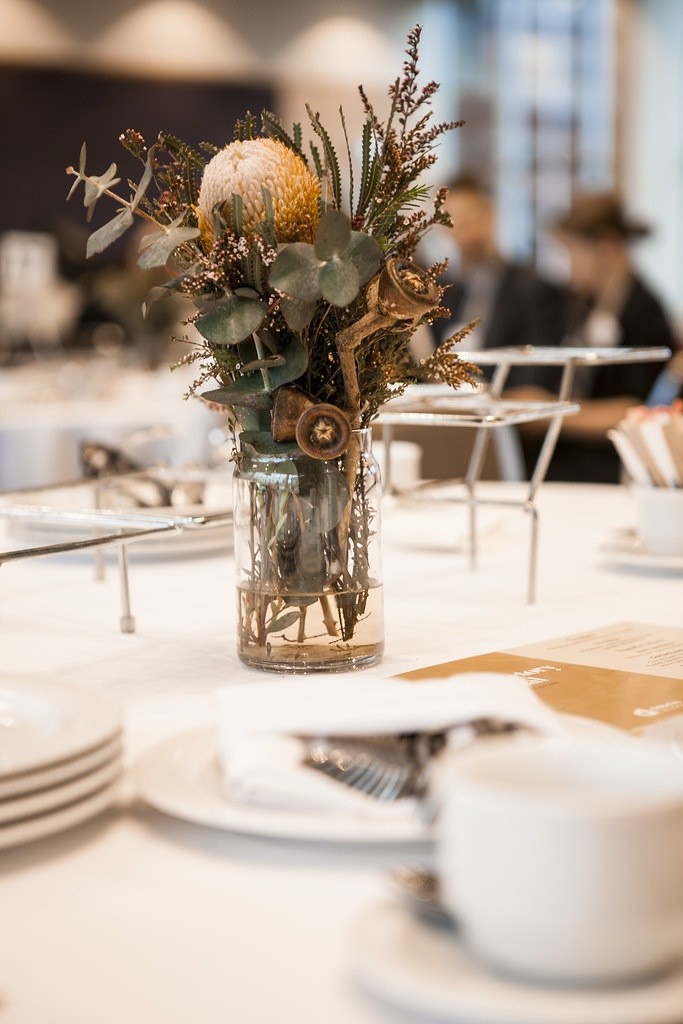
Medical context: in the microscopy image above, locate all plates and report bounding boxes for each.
[129,699,515,843]
[348,889,683,1023]
[0,668,127,851]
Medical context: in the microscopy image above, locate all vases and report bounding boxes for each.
[230,425,384,682]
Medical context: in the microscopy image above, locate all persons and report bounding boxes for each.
[408,174,569,406]
[523,196,677,480]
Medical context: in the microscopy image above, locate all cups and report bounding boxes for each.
[432,731,683,985]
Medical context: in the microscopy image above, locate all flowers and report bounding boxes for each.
[64,21,485,461]
